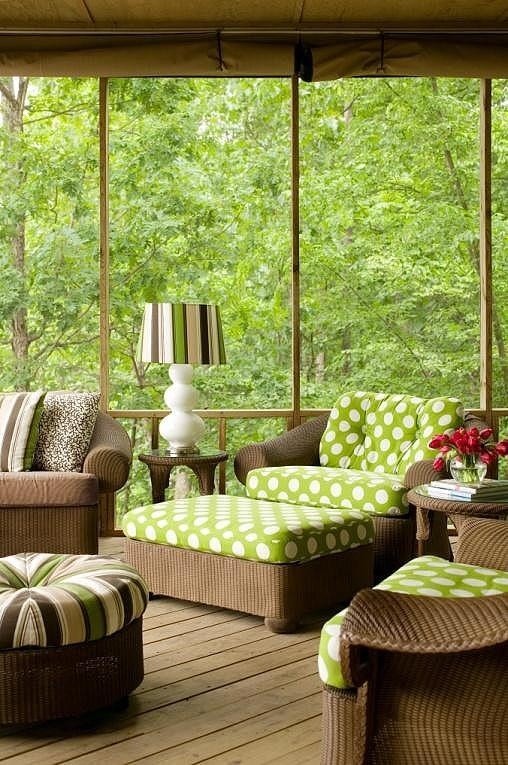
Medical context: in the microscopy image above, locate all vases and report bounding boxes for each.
[449,453,488,484]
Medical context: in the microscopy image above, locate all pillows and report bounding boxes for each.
[32,390,102,472]
[320,392,462,477]
[1,393,48,471]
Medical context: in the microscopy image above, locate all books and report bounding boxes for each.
[426,484,507,500]
[430,476,508,495]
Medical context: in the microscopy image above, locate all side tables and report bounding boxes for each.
[138,447,229,503]
[407,479,508,556]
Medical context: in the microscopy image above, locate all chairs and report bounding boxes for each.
[0,410,133,555]
[234,391,488,585]
[317,515,508,765]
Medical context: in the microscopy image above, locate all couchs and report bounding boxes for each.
[118,494,376,634]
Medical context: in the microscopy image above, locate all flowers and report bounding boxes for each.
[428,426,508,473]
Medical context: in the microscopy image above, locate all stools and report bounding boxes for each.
[0,552,150,730]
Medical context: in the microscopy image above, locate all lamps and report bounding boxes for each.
[134,301,228,452]
